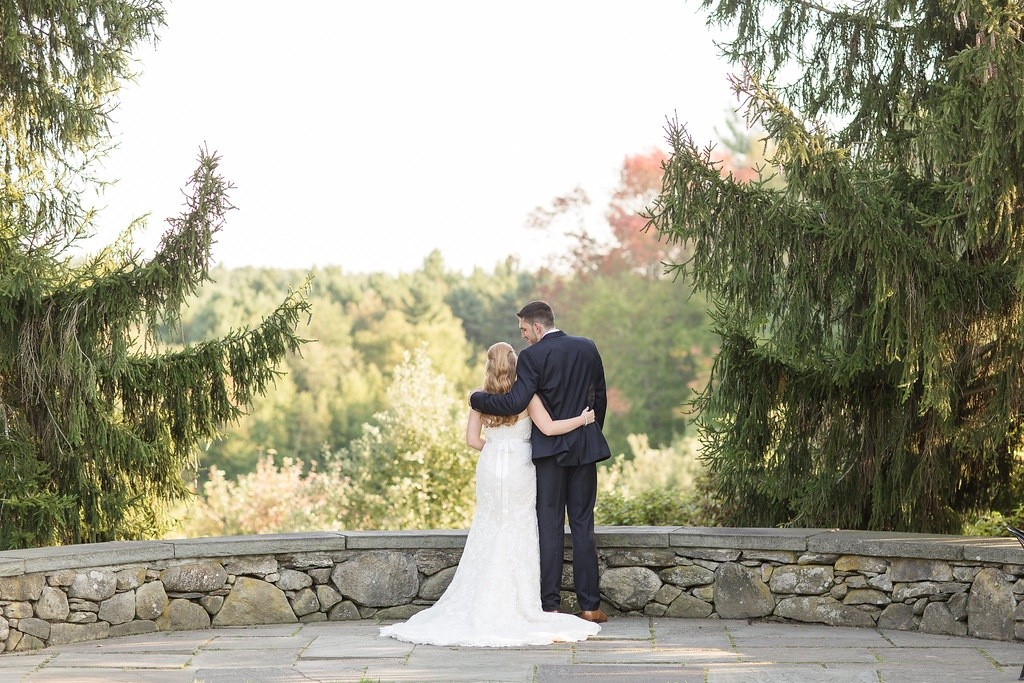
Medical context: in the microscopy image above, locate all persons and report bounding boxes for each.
[466,301,613,622]
[466,342,595,626]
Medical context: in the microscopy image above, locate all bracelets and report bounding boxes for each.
[582,413,588,427]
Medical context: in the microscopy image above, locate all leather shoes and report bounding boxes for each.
[545,610,560,613]
[582,609,608,623]
